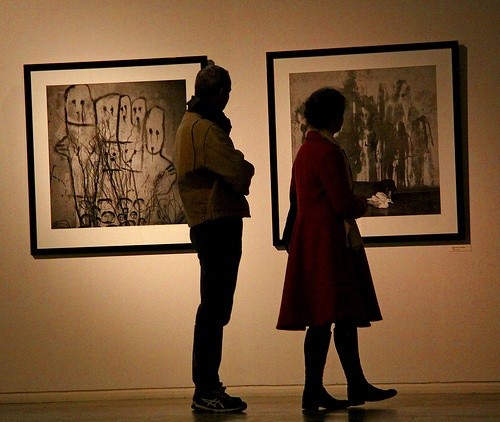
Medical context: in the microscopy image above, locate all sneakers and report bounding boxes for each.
[190,388,250,416]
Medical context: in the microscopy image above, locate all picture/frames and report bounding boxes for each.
[265,40,468,247]
[23,56,207,256]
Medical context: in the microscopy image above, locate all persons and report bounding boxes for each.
[276,83,397,412]
[177,62,254,412]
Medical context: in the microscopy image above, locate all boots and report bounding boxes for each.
[334,328,398,407]
[301,328,351,412]
